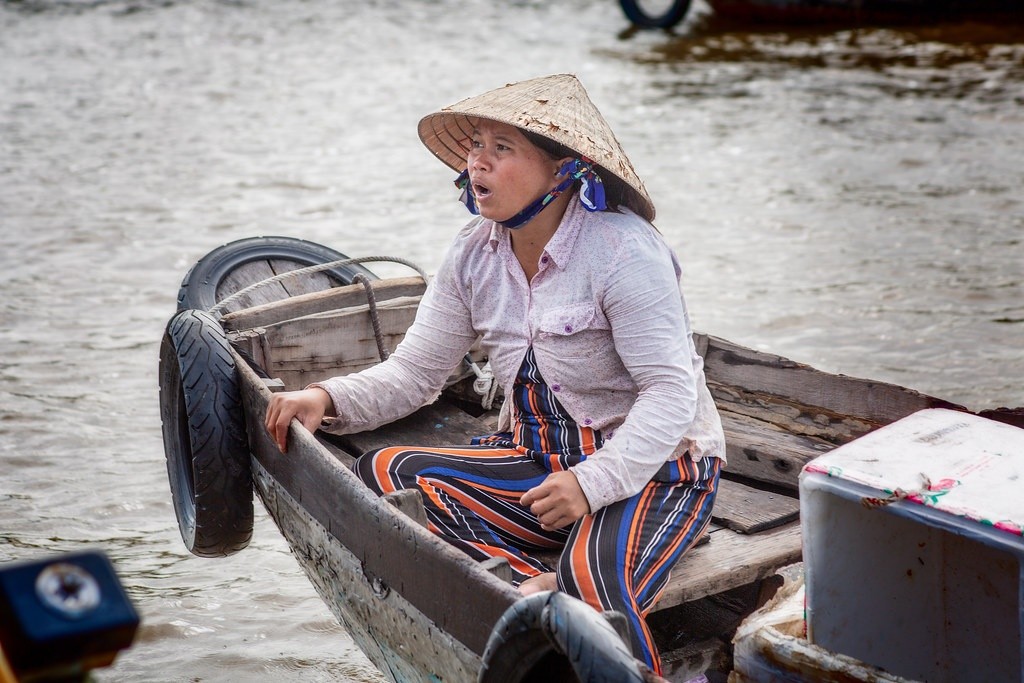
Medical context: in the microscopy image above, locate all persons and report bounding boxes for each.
[261,70,728,680]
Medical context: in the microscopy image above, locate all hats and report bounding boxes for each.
[418,73,656,225]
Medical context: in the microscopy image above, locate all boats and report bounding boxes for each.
[159,235,1024,683]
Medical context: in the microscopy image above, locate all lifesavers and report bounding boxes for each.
[175,233,385,321]
[155,307,256,559]
[472,588,646,683]
